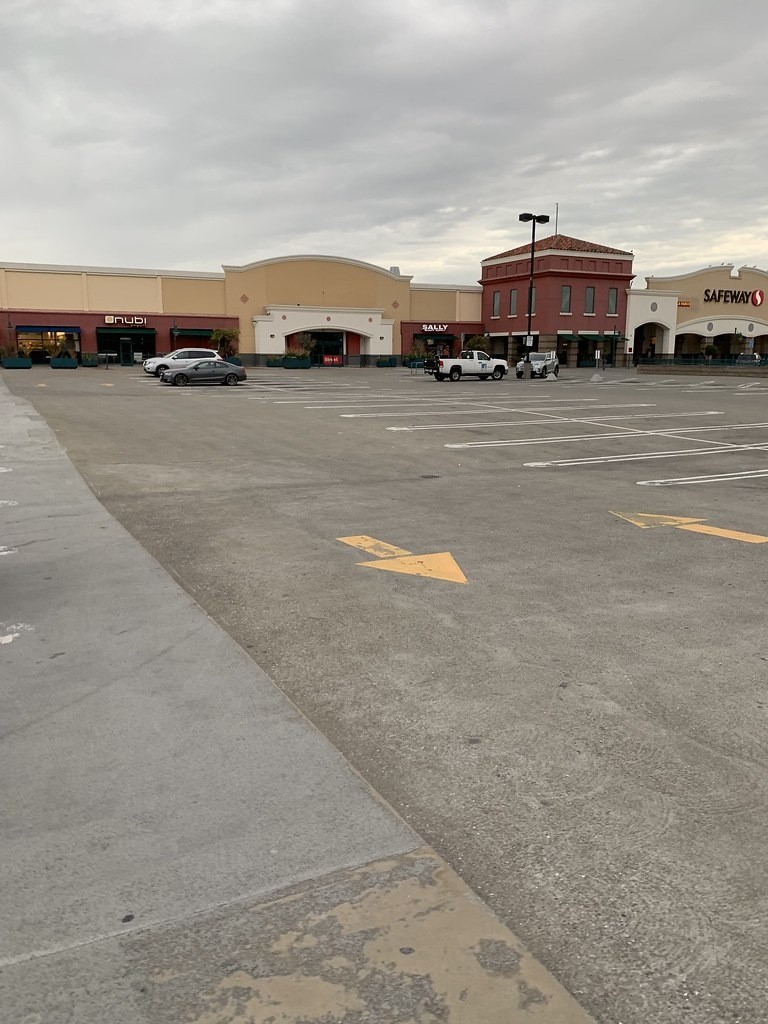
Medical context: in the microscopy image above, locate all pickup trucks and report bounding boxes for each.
[423,350,509,382]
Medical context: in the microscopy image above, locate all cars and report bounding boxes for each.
[736,354,758,366]
[159,358,247,386]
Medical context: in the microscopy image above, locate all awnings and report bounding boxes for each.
[559,334,629,342]
[414,332,457,341]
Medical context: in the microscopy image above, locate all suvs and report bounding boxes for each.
[142,348,224,378]
[515,352,559,379]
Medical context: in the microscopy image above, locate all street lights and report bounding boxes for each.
[519,212,550,363]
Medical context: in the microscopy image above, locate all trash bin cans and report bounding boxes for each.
[134,352,142,361]
